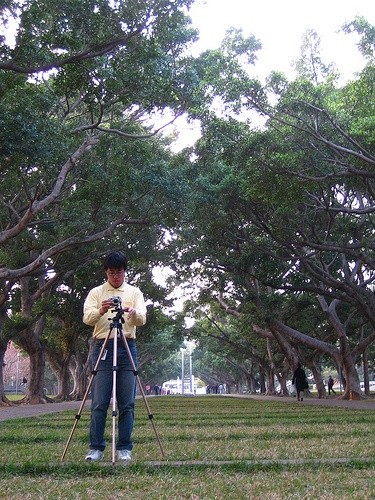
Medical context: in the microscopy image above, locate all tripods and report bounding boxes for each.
[61,307,165,466]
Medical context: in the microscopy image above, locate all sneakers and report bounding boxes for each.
[85,449,104,461]
[117,449,132,461]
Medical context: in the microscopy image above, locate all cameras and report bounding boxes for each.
[108,296,122,308]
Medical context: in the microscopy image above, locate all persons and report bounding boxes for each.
[327,376,336,395]
[291,363,306,401]
[145,384,224,395]
[83,250,146,463]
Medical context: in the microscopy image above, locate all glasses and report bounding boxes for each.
[107,270,125,276]
